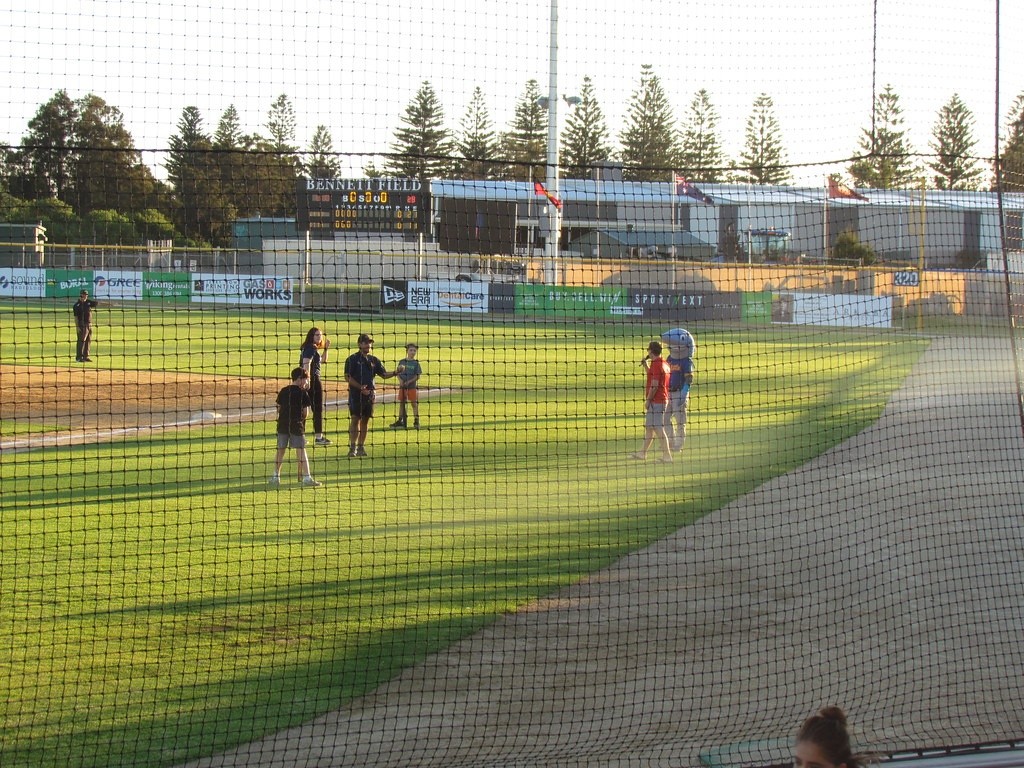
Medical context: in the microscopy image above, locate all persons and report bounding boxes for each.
[389,344,422,430]
[265,368,323,487]
[297,327,334,445]
[631,328,696,465]
[343,333,406,458]
[73,289,114,363]
[790,707,855,768]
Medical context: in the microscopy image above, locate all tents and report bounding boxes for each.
[429,179,1024,273]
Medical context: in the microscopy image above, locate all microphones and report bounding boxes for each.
[641,355,650,365]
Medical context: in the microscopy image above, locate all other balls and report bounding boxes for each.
[396,365,406,372]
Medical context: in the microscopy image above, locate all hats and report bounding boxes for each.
[81,289,89,295]
[358,334,375,344]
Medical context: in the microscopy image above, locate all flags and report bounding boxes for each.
[828,177,870,203]
[534,175,562,209]
[674,173,715,206]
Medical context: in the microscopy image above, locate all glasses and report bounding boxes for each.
[315,334,321,336]
[647,347,651,350]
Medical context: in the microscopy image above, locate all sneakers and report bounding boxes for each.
[390,421,405,428]
[305,478,322,486]
[76,357,84,362]
[269,477,281,484]
[348,449,356,457]
[415,421,420,427]
[316,436,333,445]
[357,448,367,456]
[86,356,93,362]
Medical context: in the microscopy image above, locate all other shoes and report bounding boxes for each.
[632,452,647,460]
[672,446,683,452]
[657,457,674,464]
